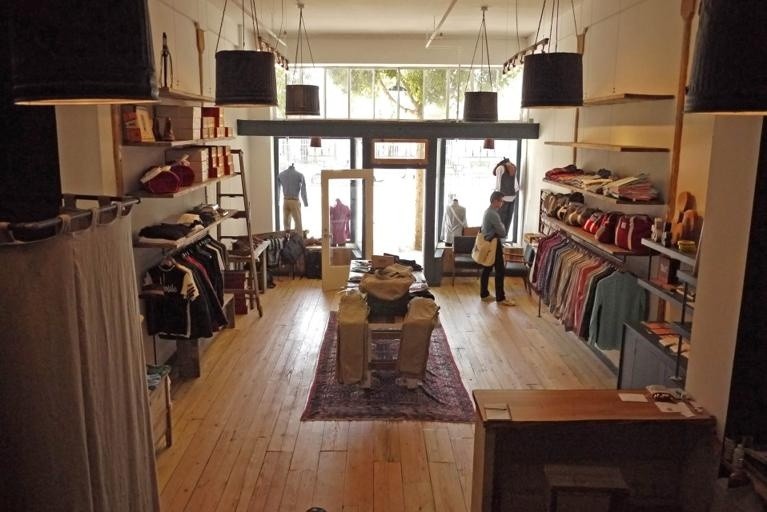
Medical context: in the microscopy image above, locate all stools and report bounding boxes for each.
[545,464,629,512]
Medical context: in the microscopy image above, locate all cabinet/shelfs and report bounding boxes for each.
[149,373,172,447]
[542,93,675,256]
[637,238,696,342]
[120,88,237,249]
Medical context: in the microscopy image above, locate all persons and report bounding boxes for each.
[494,156,520,237]
[329,199,353,247]
[673,191,703,243]
[479,192,515,305]
[440,198,468,247]
[275,162,308,241]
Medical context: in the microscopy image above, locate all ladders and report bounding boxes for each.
[216,149,263,318]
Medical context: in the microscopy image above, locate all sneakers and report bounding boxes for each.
[482,294,496,301]
[146,363,171,388]
[497,300,515,305]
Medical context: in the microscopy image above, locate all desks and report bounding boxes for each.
[227,241,271,309]
[617,321,691,390]
[158,293,235,378]
[345,261,447,388]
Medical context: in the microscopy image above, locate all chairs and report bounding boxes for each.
[452,228,532,296]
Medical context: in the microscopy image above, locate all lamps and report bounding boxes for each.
[522,0,584,108]
[216,0,277,108]
[6,0,161,106]
[483,139,494,149]
[464,7,498,123]
[311,137,321,147]
[683,0,766,116]
[286,4,319,115]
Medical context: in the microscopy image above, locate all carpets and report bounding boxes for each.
[301,311,476,424]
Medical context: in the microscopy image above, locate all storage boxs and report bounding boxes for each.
[155,105,234,182]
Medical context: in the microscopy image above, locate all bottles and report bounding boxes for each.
[731,444,744,468]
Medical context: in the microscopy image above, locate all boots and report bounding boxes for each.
[154,118,161,140]
[162,118,175,141]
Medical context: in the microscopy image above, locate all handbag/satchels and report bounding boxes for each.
[543,192,653,251]
[471,232,497,267]
[362,265,413,301]
